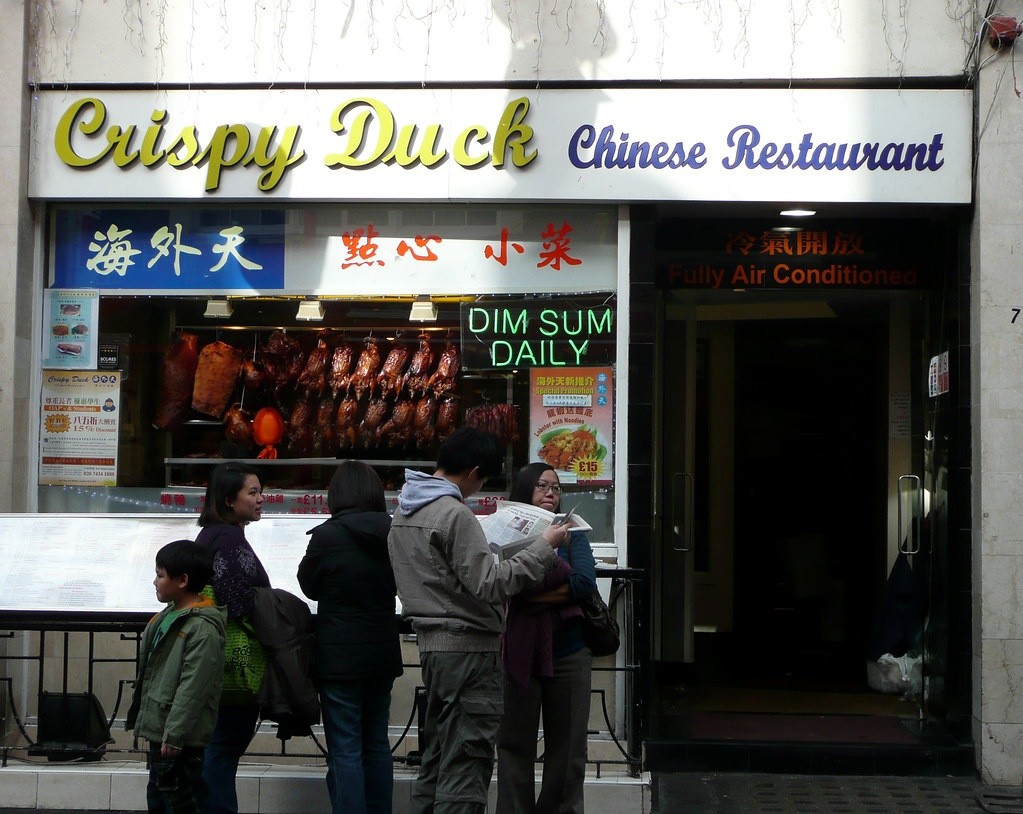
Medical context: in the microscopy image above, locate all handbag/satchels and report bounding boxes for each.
[583,596,621,657]
[200,585,264,693]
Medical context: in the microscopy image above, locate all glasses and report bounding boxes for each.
[534,482,563,495]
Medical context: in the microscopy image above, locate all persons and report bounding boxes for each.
[298,461,404,814]
[124,541,229,814]
[387,427,574,814]
[192,462,273,814]
[494,462,597,814]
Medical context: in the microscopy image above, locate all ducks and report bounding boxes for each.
[462,403,518,441]
[224,327,459,450]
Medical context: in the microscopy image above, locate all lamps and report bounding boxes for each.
[409,295,439,323]
[295,295,325,321]
[203,295,234,319]
